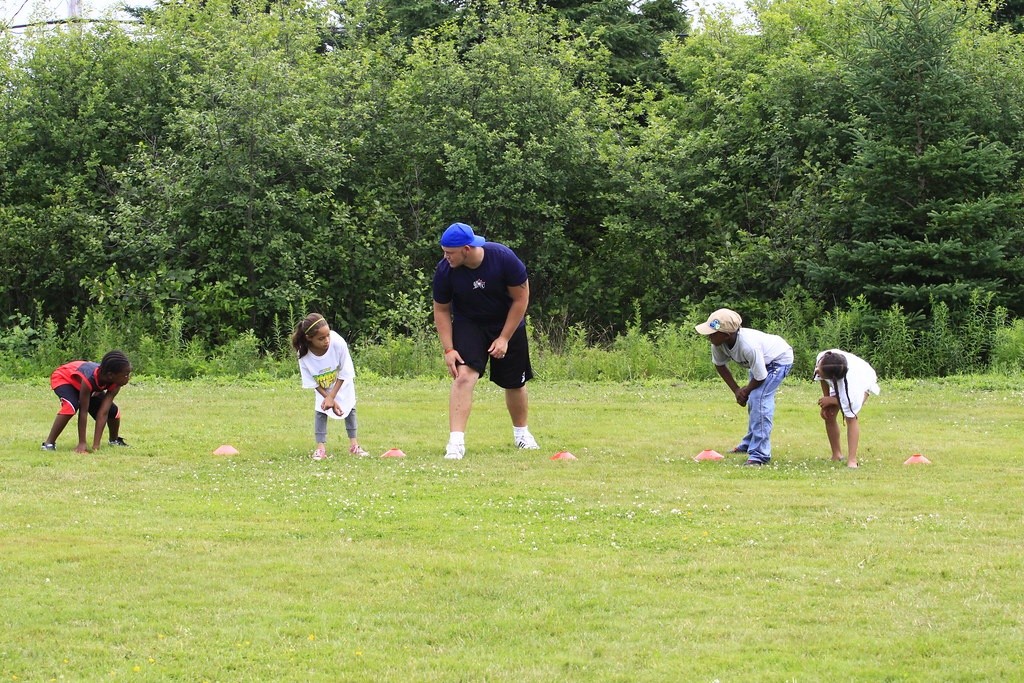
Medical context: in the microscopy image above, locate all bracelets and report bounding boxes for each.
[444,347,454,354]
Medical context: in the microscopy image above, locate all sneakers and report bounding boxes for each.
[444,444,465,459]
[350,445,369,457]
[514,435,539,450]
[312,449,325,461]
[743,460,768,467]
[728,448,747,454]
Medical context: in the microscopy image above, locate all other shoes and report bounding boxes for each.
[41,442,57,451]
[108,438,131,447]
[829,456,859,469]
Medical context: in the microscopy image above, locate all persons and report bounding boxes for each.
[811,348,881,469]
[695,308,794,466]
[432,223,539,459]
[290,312,369,461]
[40,350,131,455]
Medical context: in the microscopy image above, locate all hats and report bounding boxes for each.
[695,309,742,335]
[440,223,485,248]
